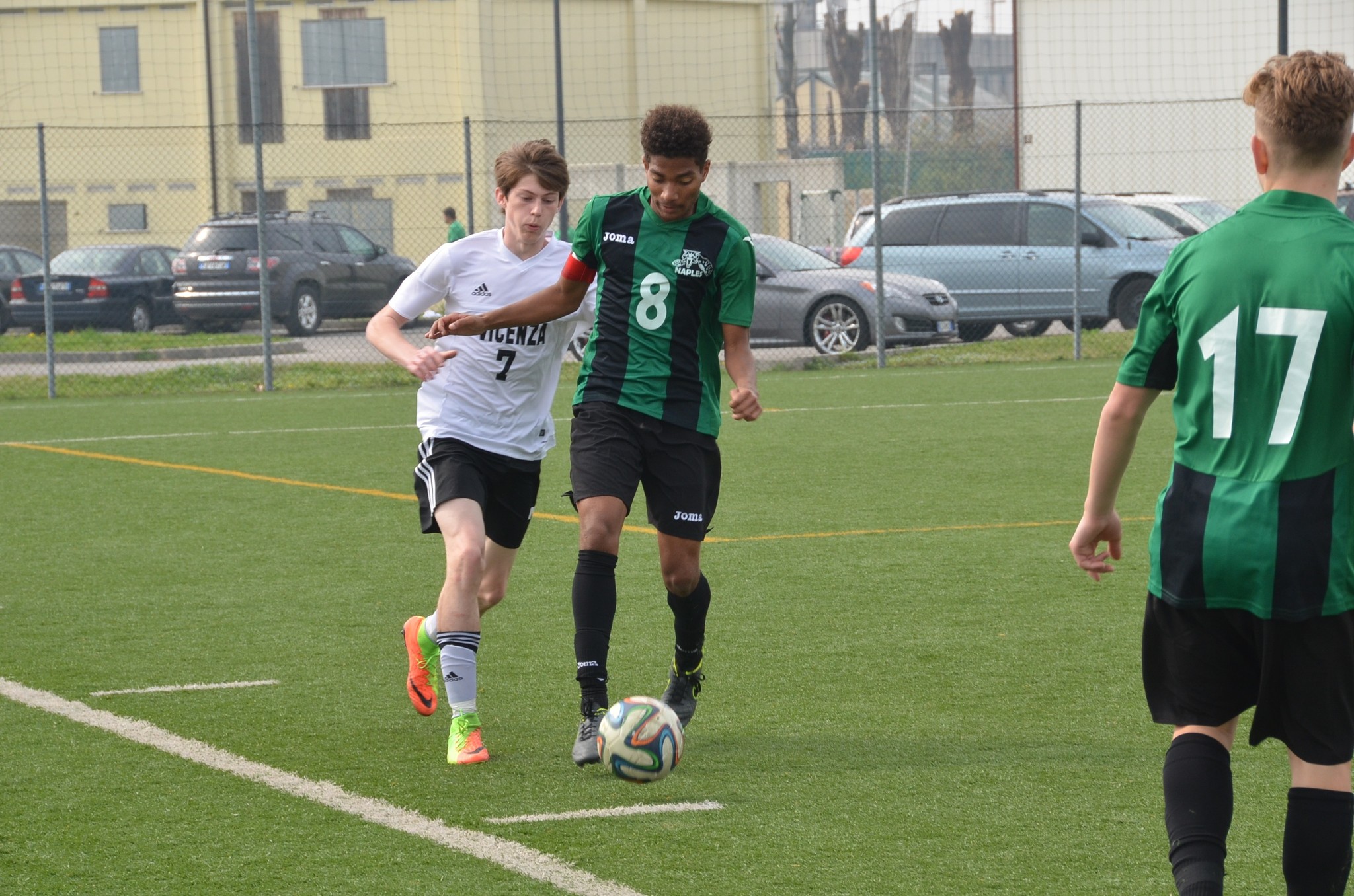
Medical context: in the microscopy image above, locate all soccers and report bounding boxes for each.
[598,695,685,783]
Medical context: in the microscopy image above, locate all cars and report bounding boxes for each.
[0,244,52,334]
[8,244,246,335]
[1083,191,1237,238]
[568,233,961,364]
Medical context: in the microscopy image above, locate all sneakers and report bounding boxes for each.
[572,698,608,767]
[660,646,705,729]
[402,616,439,717]
[447,709,489,765]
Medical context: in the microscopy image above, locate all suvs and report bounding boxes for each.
[834,185,1193,344]
[171,207,422,337]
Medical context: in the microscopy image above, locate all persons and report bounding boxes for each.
[443,207,465,243]
[1068,49,1354,896]
[425,103,763,766]
[364,136,598,767]
